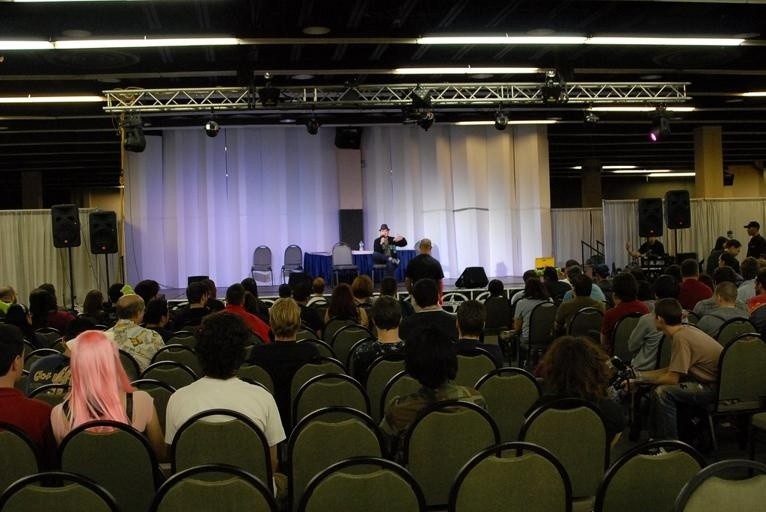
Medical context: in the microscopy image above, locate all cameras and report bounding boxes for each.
[611,354,636,391]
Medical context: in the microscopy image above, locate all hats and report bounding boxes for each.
[744,221,760,229]
[379,224,390,231]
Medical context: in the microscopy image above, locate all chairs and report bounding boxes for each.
[2,473,122,510]
[405,401,497,508]
[371,261,394,290]
[520,397,616,502]
[598,443,700,509]
[170,407,273,482]
[288,409,387,505]
[154,461,273,510]
[331,240,360,289]
[301,455,427,510]
[682,331,766,463]
[0,34,763,52]
[251,245,274,288]
[58,418,157,512]
[677,459,765,511]
[2,267,766,437]
[448,441,573,510]
[1,423,39,489]
[280,245,305,286]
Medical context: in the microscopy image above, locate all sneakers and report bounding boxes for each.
[392,258,400,265]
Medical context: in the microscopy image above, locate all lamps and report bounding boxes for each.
[118,102,672,153]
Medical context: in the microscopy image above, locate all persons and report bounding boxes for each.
[726,230,735,241]
[703,236,728,270]
[720,240,742,274]
[625,232,666,257]
[2,278,324,476]
[744,220,766,259]
[325,273,511,380]
[403,236,445,307]
[373,224,409,282]
[380,324,487,442]
[512,258,765,456]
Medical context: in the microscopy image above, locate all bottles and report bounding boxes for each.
[359,241,364,252]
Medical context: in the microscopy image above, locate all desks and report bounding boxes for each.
[304,248,417,288]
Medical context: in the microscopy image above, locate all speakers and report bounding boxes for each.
[455,267,489,289]
[52,204,81,248]
[188,276,209,286]
[664,189,691,229]
[639,198,663,237]
[293,279,313,302]
[677,252,696,265]
[89,211,118,254]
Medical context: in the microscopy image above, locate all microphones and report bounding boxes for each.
[382,235,386,249]
